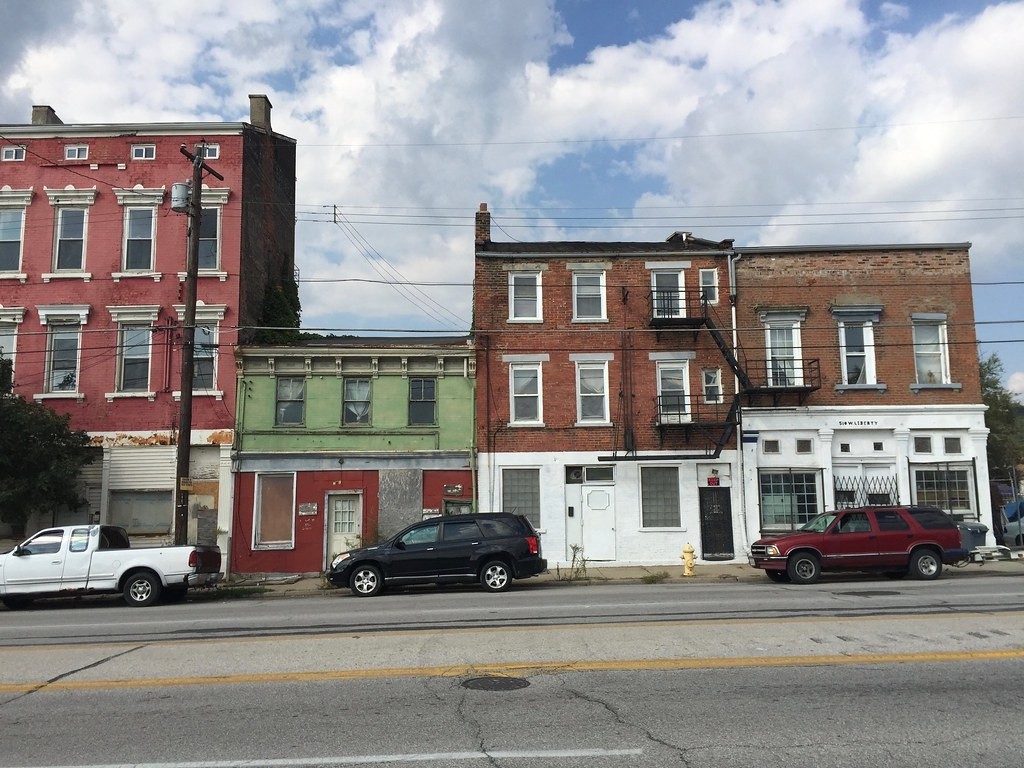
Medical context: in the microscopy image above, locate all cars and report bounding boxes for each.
[1001,515,1024,547]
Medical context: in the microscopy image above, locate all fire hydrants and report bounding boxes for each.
[678,542,698,577]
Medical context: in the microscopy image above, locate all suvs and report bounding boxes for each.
[326,509,547,598]
[747,503,974,584]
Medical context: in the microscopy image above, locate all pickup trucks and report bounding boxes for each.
[0,523,225,608]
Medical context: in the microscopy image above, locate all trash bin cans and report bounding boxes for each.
[960,522,989,563]
